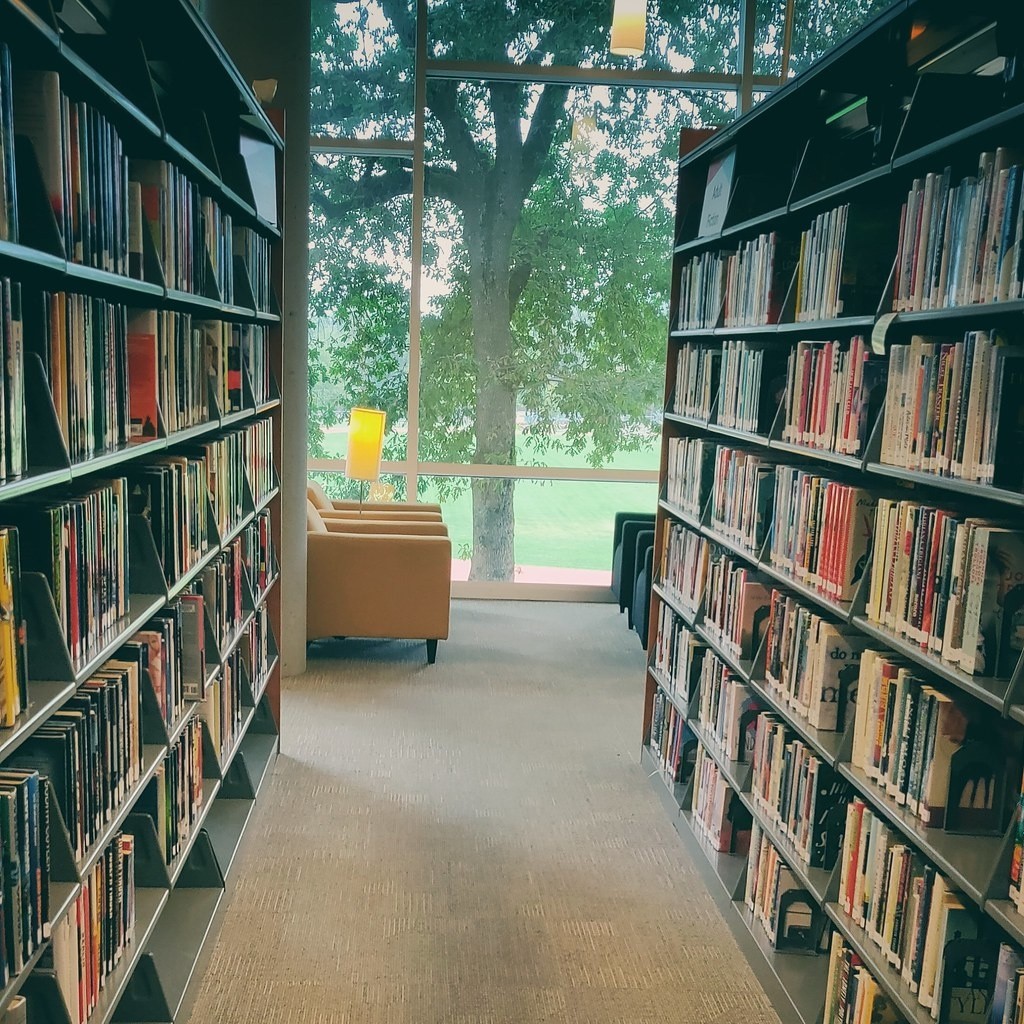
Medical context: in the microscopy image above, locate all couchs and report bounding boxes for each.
[631,529,655,651]
[609,511,656,631]
[306,497,452,664]
[306,479,443,522]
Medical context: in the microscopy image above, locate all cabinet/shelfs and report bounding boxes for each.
[639,0,1024,1024]
[0,0,288,1024]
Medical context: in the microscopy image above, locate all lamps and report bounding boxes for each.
[609,0,647,58]
[345,407,386,520]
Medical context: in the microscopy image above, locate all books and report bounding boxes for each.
[0,70,273,1024]
[650,145,1024,1024]
